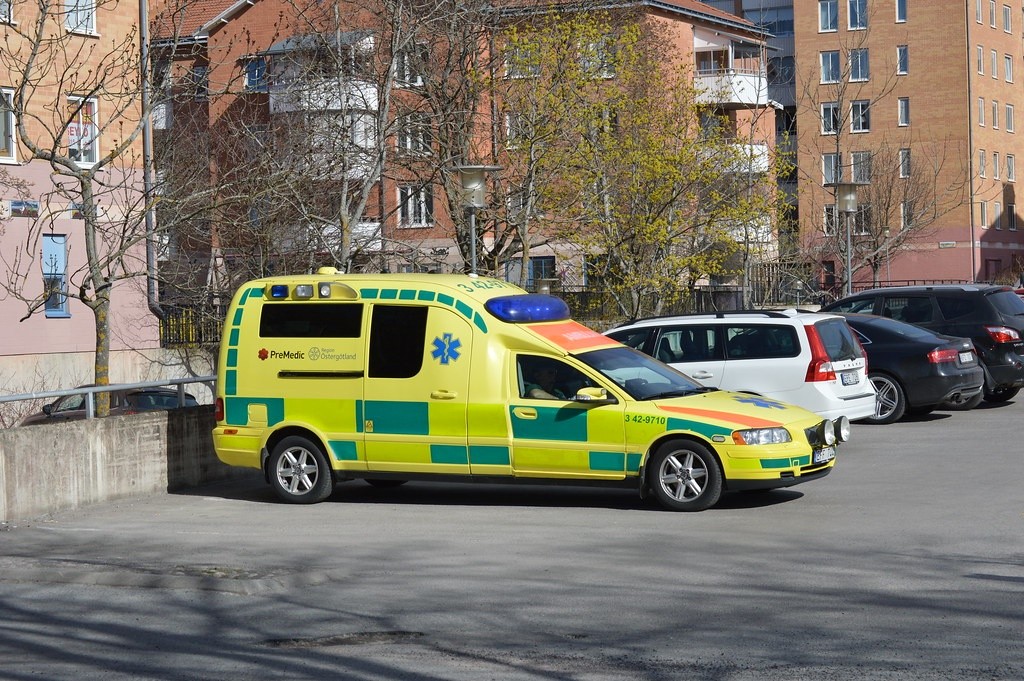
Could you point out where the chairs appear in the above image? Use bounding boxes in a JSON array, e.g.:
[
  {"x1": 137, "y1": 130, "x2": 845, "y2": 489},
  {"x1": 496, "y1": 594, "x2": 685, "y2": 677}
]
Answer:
[
  {"x1": 884, "y1": 307, "x2": 892, "y2": 317},
  {"x1": 658, "y1": 330, "x2": 698, "y2": 363},
  {"x1": 899, "y1": 305, "x2": 913, "y2": 323}
]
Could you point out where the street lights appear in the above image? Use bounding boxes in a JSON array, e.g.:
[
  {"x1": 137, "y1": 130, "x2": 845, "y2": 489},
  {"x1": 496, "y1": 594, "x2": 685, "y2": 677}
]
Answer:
[
  {"x1": 878, "y1": 226, "x2": 894, "y2": 286},
  {"x1": 443, "y1": 165, "x2": 504, "y2": 275},
  {"x1": 792, "y1": 280, "x2": 805, "y2": 308},
  {"x1": 822, "y1": 181, "x2": 873, "y2": 297}
]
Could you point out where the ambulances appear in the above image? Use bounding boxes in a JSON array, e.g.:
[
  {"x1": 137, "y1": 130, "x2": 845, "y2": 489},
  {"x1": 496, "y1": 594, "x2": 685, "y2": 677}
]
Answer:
[{"x1": 208, "y1": 266, "x2": 853, "y2": 515}]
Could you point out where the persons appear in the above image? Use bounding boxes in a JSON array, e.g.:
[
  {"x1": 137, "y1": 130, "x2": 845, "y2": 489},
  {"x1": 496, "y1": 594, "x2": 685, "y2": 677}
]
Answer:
[{"x1": 526, "y1": 365, "x2": 566, "y2": 400}]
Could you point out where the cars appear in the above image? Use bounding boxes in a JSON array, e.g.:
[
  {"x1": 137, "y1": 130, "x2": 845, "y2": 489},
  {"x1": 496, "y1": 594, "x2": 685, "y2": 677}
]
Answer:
[
  {"x1": 826, "y1": 312, "x2": 984, "y2": 424},
  {"x1": 812, "y1": 284, "x2": 1024, "y2": 411},
  {"x1": 599, "y1": 306, "x2": 877, "y2": 422}
]
